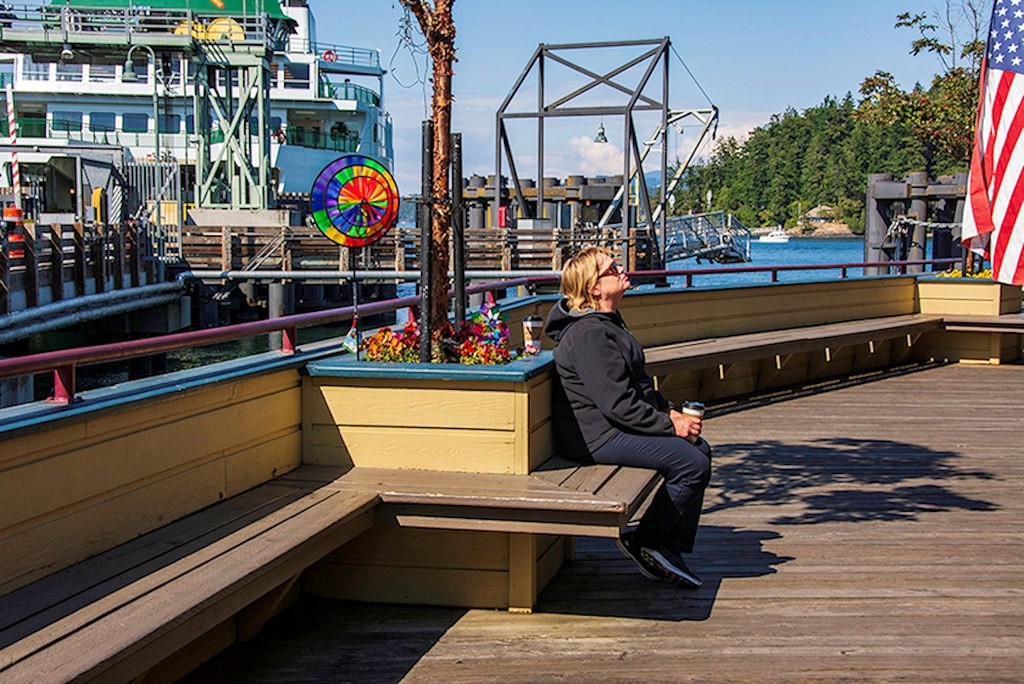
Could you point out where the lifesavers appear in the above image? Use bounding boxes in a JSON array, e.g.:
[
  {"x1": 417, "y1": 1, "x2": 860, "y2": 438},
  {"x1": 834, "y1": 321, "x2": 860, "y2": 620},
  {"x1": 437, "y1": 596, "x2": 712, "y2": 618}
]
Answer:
[{"x1": 324, "y1": 51, "x2": 334, "y2": 61}]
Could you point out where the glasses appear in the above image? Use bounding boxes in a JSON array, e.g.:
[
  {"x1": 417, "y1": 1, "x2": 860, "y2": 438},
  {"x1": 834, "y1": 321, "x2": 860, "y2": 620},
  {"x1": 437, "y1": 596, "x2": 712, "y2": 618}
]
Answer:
[{"x1": 599, "y1": 260, "x2": 619, "y2": 278}]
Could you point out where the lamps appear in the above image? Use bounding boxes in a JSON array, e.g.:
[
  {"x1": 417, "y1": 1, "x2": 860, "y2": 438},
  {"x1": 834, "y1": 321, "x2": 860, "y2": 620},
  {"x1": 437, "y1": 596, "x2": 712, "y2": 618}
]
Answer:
[
  {"x1": 60, "y1": 44, "x2": 75, "y2": 61},
  {"x1": 594, "y1": 123, "x2": 610, "y2": 144}
]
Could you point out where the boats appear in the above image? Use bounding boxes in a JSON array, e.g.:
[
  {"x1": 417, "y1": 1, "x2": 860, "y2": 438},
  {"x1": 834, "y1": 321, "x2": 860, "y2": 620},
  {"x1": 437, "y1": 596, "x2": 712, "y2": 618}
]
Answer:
[
  {"x1": 1, "y1": 1, "x2": 395, "y2": 320},
  {"x1": 758, "y1": 224, "x2": 794, "y2": 243}
]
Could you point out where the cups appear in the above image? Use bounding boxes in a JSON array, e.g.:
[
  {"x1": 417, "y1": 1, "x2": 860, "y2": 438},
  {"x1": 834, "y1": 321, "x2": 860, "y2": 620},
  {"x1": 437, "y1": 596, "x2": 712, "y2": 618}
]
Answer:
[
  {"x1": 522, "y1": 315, "x2": 544, "y2": 355},
  {"x1": 681, "y1": 401, "x2": 705, "y2": 442}
]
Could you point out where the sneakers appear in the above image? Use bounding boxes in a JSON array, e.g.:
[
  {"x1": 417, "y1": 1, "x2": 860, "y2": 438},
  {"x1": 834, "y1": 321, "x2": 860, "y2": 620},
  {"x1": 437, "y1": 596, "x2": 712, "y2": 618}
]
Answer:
[
  {"x1": 640, "y1": 544, "x2": 704, "y2": 589},
  {"x1": 616, "y1": 530, "x2": 665, "y2": 580}
]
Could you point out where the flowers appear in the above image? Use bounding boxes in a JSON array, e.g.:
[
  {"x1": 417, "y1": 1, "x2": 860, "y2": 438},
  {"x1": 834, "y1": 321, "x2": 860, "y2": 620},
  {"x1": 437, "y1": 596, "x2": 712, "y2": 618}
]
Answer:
[
  {"x1": 361, "y1": 302, "x2": 512, "y2": 365},
  {"x1": 934, "y1": 267, "x2": 994, "y2": 280}
]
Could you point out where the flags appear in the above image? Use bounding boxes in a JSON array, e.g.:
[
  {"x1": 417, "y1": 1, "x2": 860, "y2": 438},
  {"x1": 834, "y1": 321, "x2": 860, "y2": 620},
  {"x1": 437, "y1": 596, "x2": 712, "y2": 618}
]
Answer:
[{"x1": 961, "y1": 0, "x2": 1024, "y2": 285}]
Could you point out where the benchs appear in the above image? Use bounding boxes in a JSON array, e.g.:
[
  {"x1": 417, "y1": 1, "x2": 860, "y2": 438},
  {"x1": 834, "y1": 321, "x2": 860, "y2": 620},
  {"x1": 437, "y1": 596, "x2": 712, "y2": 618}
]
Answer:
[
  {"x1": 642, "y1": 311, "x2": 1024, "y2": 416},
  {"x1": 0, "y1": 456, "x2": 667, "y2": 684}
]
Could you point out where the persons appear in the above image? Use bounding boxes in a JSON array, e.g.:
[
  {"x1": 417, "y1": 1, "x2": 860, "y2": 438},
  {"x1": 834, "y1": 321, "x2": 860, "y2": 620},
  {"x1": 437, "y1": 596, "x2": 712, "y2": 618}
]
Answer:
[{"x1": 544, "y1": 247, "x2": 712, "y2": 589}]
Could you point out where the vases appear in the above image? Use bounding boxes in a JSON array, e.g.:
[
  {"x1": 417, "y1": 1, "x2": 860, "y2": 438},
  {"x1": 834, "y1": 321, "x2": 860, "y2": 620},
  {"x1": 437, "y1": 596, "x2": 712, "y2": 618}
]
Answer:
[
  {"x1": 916, "y1": 275, "x2": 1023, "y2": 366},
  {"x1": 294, "y1": 349, "x2": 557, "y2": 616}
]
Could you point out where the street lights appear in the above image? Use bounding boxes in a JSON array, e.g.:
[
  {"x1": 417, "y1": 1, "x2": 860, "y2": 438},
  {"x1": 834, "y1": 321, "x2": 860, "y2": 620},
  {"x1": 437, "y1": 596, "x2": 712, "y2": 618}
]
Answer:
[{"x1": 121, "y1": 44, "x2": 163, "y2": 257}]
